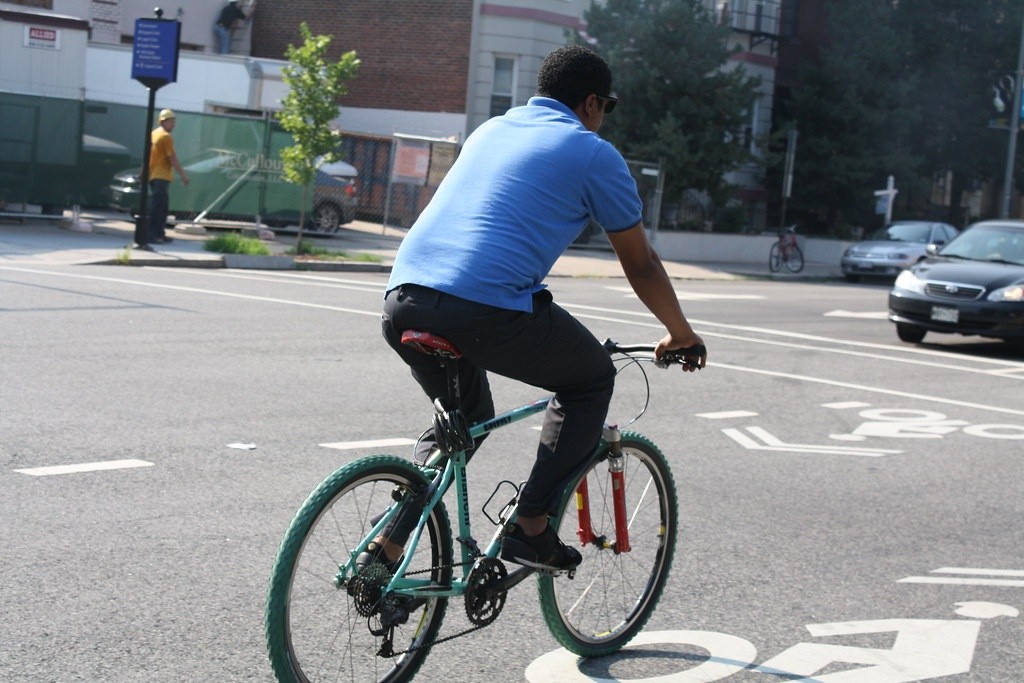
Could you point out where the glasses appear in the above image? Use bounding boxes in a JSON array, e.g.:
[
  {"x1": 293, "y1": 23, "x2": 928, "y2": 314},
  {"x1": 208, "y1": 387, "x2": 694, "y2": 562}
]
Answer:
[{"x1": 590, "y1": 94, "x2": 618, "y2": 113}]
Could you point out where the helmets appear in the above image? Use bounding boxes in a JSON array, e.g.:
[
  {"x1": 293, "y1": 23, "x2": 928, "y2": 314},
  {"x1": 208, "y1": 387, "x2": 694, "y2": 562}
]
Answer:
[{"x1": 158, "y1": 109, "x2": 175, "y2": 125}]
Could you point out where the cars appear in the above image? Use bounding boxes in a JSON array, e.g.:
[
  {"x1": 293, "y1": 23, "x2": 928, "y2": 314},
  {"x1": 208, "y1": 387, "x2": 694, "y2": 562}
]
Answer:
[
  {"x1": 888, "y1": 219, "x2": 1023, "y2": 360},
  {"x1": 840, "y1": 221, "x2": 962, "y2": 285},
  {"x1": 109, "y1": 145, "x2": 357, "y2": 238}
]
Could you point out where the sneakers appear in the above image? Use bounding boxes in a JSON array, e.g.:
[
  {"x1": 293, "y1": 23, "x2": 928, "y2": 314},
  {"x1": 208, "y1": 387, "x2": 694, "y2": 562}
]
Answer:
[
  {"x1": 356, "y1": 542, "x2": 404, "y2": 575},
  {"x1": 500, "y1": 520, "x2": 582, "y2": 571}
]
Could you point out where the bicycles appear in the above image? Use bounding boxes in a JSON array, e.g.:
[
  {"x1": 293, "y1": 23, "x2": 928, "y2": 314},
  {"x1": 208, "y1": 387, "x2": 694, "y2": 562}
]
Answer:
[
  {"x1": 263, "y1": 329, "x2": 706, "y2": 683},
  {"x1": 769, "y1": 224, "x2": 805, "y2": 273}
]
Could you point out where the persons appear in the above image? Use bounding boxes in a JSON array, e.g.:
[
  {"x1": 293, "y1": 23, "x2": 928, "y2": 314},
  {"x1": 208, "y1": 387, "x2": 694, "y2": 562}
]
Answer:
[
  {"x1": 135, "y1": 107, "x2": 189, "y2": 244},
  {"x1": 214, "y1": 1, "x2": 256, "y2": 56},
  {"x1": 352, "y1": 44, "x2": 706, "y2": 591}
]
